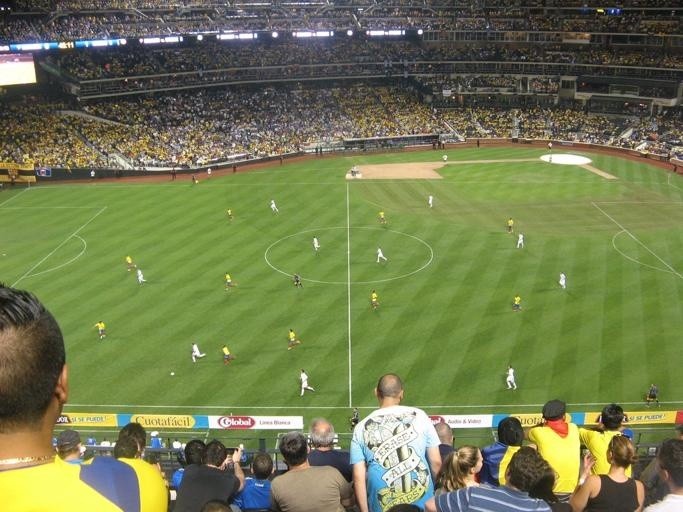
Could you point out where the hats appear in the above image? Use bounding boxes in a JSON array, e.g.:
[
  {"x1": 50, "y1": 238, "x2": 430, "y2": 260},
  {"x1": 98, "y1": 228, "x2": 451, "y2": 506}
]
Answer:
[
  {"x1": 56, "y1": 430, "x2": 80, "y2": 450},
  {"x1": 542, "y1": 400, "x2": 566, "y2": 420}
]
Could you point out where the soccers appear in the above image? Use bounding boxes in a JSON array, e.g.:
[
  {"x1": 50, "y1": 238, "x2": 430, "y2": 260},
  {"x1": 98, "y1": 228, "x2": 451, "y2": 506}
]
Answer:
[{"x1": 170, "y1": 371, "x2": 175, "y2": 376}]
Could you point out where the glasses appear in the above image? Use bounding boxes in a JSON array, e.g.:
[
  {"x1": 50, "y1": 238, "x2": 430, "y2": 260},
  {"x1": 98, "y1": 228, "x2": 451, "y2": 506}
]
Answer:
[{"x1": 611, "y1": 434, "x2": 630, "y2": 448}]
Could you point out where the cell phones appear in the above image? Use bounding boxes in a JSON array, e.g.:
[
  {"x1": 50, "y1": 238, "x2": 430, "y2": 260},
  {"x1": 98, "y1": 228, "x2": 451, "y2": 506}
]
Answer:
[{"x1": 225, "y1": 447, "x2": 239, "y2": 455}]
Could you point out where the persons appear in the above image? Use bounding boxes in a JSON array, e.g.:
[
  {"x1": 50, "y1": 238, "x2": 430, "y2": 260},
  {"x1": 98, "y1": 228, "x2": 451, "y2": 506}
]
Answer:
[
  {"x1": 125, "y1": 254, "x2": 135, "y2": 269},
  {"x1": 55, "y1": 408, "x2": 422, "y2": 511},
  {"x1": 504, "y1": 364, "x2": 517, "y2": 389},
  {"x1": 512, "y1": 294, "x2": 522, "y2": 311},
  {"x1": 221, "y1": 343, "x2": 238, "y2": 366},
  {"x1": 645, "y1": 383, "x2": 660, "y2": 408},
  {"x1": 287, "y1": 328, "x2": 301, "y2": 352},
  {"x1": 292, "y1": 273, "x2": 304, "y2": 290},
  {"x1": 427, "y1": 194, "x2": 433, "y2": 208},
  {"x1": 558, "y1": 271, "x2": 566, "y2": 290},
  {"x1": 225, "y1": 207, "x2": 233, "y2": 219},
  {"x1": 349, "y1": 374, "x2": 442, "y2": 512},
  {"x1": 0, "y1": 280, "x2": 168, "y2": 512},
  {"x1": 93, "y1": 321, "x2": 108, "y2": 339},
  {"x1": 507, "y1": 217, "x2": 513, "y2": 232},
  {"x1": 516, "y1": 233, "x2": 524, "y2": 249},
  {"x1": 1, "y1": 1, "x2": 682, "y2": 184},
  {"x1": 134, "y1": 267, "x2": 146, "y2": 286},
  {"x1": 375, "y1": 247, "x2": 388, "y2": 264},
  {"x1": 377, "y1": 209, "x2": 387, "y2": 226},
  {"x1": 221, "y1": 271, "x2": 238, "y2": 293},
  {"x1": 310, "y1": 235, "x2": 322, "y2": 252},
  {"x1": 425, "y1": 400, "x2": 682, "y2": 511},
  {"x1": 268, "y1": 198, "x2": 280, "y2": 216},
  {"x1": 298, "y1": 370, "x2": 315, "y2": 398},
  {"x1": 190, "y1": 343, "x2": 206, "y2": 365},
  {"x1": 370, "y1": 290, "x2": 378, "y2": 310}
]
